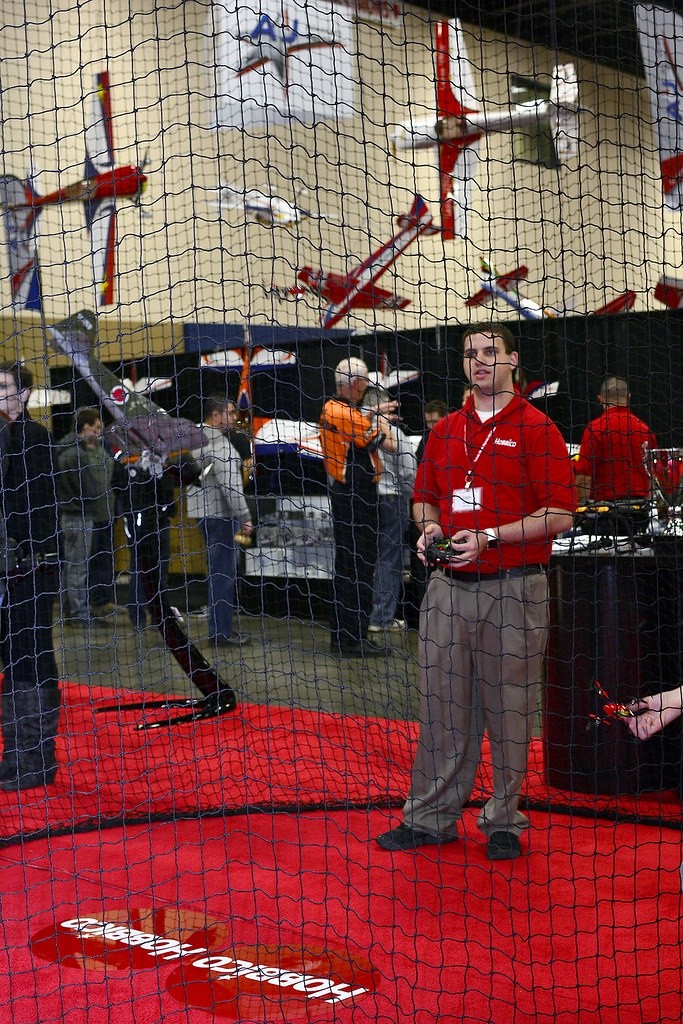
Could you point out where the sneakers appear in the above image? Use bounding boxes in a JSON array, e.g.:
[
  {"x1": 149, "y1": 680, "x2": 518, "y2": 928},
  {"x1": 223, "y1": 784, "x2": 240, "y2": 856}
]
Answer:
[
  {"x1": 377, "y1": 822, "x2": 458, "y2": 849},
  {"x1": 488, "y1": 831, "x2": 520, "y2": 859}
]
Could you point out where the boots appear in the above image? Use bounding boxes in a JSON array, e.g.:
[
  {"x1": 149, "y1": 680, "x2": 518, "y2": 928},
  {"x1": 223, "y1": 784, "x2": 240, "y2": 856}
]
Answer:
[{"x1": 0, "y1": 678, "x2": 61, "y2": 791}]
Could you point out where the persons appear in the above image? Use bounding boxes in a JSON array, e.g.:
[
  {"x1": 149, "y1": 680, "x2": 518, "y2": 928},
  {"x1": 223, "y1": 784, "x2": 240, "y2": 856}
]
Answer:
[
  {"x1": 461, "y1": 384, "x2": 472, "y2": 407},
  {"x1": 409, "y1": 400, "x2": 448, "y2": 631},
  {"x1": 623, "y1": 686, "x2": 683, "y2": 740},
  {"x1": 185, "y1": 397, "x2": 253, "y2": 646},
  {"x1": 577, "y1": 376, "x2": 658, "y2": 537},
  {"x1": 113, "y1": 452, "x2": 200, "y2": 630},
  {"x1": 0, "y1": 360, "x2": 60, "y2": 788},
  {"x1": 322, "y1": 357, "x2": 404, "y2": 660},
  {"x1": 375, "y1": 322, "x2": 576, "y2": 858},
  {"x1": 58, "y1": 407, "x2": 129, "y2": 628},
  {"x1": 362, "y1": 390, "x2": 420, "y2": 632}
]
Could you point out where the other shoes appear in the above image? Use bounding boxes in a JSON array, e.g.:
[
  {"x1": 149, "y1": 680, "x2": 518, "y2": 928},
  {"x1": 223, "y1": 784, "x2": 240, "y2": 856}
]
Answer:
[
  {"x1": 341, "y1": 646, "x2": 391, "y2": 657},
  {"x1": 368, "y1": 619, "x2": 405, "y2": 631},
  {"x1": 90, "y1": 601, "x2": 127, "y2": 617},
  {"x1": 331, "y1": 640, "x2": 376, "y2": 653},
  {"x1": 208, "y1": 633, "x2": 253, "y2": 646}
]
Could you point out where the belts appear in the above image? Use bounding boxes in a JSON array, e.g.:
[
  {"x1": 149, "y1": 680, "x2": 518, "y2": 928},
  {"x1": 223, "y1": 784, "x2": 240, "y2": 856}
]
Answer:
[{"x1": 436, "y1": 564, "x2": 544, "y2": 582}]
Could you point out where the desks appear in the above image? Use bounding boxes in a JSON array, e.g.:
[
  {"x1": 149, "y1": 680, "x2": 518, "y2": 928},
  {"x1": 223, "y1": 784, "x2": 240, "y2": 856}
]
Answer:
[
  {"x1": 241, "y1": 454, "x2": 427, "y2": 630},
  {"x1": 543, "y1": 536, "x2": 682, "y2": 795}
]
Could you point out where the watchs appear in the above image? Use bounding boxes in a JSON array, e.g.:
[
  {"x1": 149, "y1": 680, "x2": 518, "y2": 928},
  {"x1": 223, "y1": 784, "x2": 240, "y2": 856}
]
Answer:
[{"x1": 485, "y1": 529, "x2": 498, "y2": 550}]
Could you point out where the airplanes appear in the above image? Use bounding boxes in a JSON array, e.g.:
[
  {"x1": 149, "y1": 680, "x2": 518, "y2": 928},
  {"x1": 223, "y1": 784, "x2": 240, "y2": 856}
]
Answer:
[
  {"x1": 231, "y1": 7, "x2": 349, "y2": 99},
  {"x1": 11, "y1": 70, "x2": 149, "y2": 308},
  {"x1": 462, "y1": 255, "x2": 639, "y2": 323},
  {"x1": 191, "y1": 323, "x2": 585, "y2": 497},
  {"x1": 587, "y1": 677, "x2": 648, "y2": 729},
  {"x1": 391, "y1": 17, "x2": 582, "y2": 241},
  {"x1": 288, "y1": 193, "x2": 444, "y2": 330},
  {"x1": 44, "y1": 309, "x2": 209, "y2": 493},
  {"x1": 209, "y1": 180, "x2": 340, "y2": 231}
]
